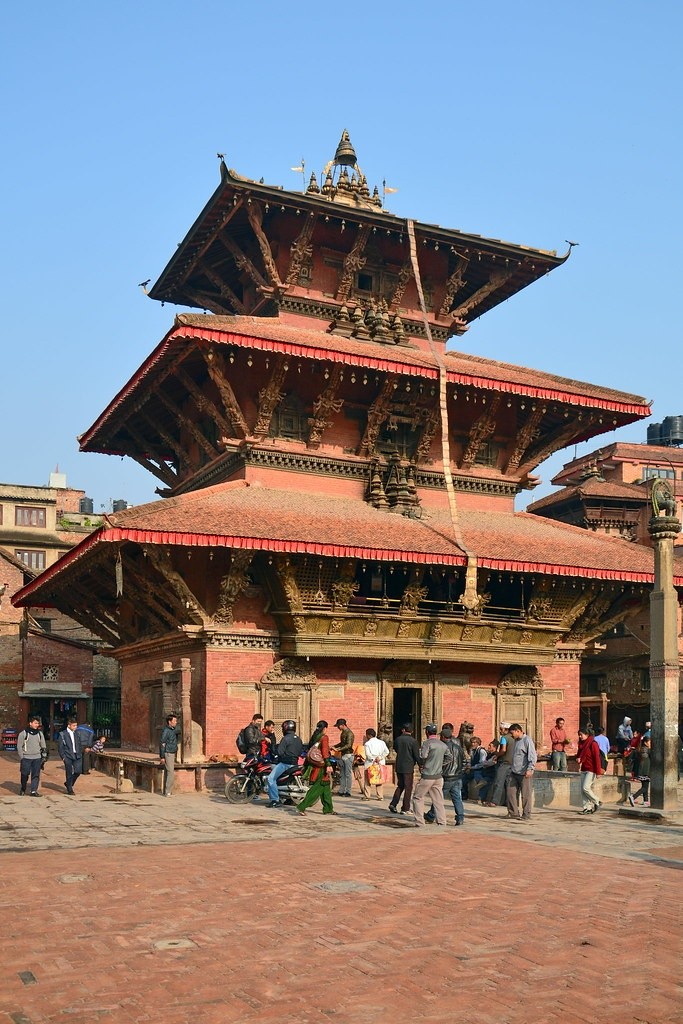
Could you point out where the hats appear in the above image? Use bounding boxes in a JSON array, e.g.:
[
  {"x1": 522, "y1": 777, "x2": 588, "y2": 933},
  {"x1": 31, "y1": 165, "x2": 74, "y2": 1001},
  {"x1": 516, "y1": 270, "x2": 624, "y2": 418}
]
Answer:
[
  {"x1": 423, "y1": 725, "x2": 437, "y2": 734},
  {"x1": 500, "y1": 722, "x2": 512, "y2": 729},
  {"x1": 439, "y1": 729, "x2": 452, "y2": 738},
  {"x1": 334, "y1": 719, "x2": 346, "y2": 727}
]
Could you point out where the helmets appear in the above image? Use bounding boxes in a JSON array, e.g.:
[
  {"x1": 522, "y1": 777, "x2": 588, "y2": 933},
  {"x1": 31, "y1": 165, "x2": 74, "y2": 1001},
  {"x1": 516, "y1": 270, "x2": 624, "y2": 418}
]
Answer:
[{"x1": 281, "y1": 720, "x2": 297, "y2": 736}]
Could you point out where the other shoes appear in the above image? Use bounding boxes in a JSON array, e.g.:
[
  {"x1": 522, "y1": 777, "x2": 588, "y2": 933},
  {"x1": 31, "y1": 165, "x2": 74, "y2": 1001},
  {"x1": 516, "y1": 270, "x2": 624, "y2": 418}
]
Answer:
[
  {"x1": 629, "y1": 796, "x2": 635, "y2": 807},
  {"x1": 578, "y1": 809, "x2": 592, "y2": 815},
  {"x1": 336, "y1": 792, "x2": 344, "y2": 796},
  {"x1": 67, "y1": 788, "x2": 76, "y2": 795},
  {"x1": 325, "y1": 811, "x2": 338, "y2": 815},
  {"x1": 389, "y1": 805, "x2": 397, "y2": 813},
  {"x1": 295, "y1": 807, "x2": 307, "y2": 816},
  {"x1": 502, "y1": 814, "x2": 520, "y2": 819},
  {"x1": 361, "y1": 796, "x2": 370, "y2": 801},
  {"x1": 20, "y1": 789, "x2": 25, "y2": 796},
  {"x1": 518, "y1": 816, "x2": 530, "y2": 821},
  {"x1": 64, "y1": 782, "x2": 68, "y2": 787},
  {"x1": 378, "y1": 797, "x2": 383, "y2": 801},
  {"x1": 253, "y1": 795, "x2": 262, "y2": 801},
  {"x1": 593, "y1": 801, "x2": 603, "y2": 814},
  {"x1": 84, "y1": 772, "x2": 90, "y2": 775},
  {"x1": 30, "y1": 792, "x2": 43, "y2": 797},
  {"x1": 462, "y1": 797, "x2": 468, "y2": 800},
  {"x1": 642, "y1": 801, "x2": 649, "y2": 806},
  {"x1": 424, "y1": 812, "x2": 434, "y2": 823},
  {"x1": 400, "y1": 809, "x2": 414, "y2": 815},
  {"x1": 345, "y1": 793, "x2": 351, "y2": 797},
  {"x1": 163, "y1": 793, "x2": 176, "y2": 797},
  {"x1": 266, "y1": 800, "x2": 283, "y2": 808},
  {"x1": 473, "y1": 780, "x2": 487, "y2": 790},
  {"x1": 473, "y1": 800, "x2": 486, "y2": 804},
  {"x1": 455, "y1": 821, "x2": 463, "y2": 826},
  {"x1": 284, "y1": 799, "x2": 293, "y2": 805},
  {"x1": 484, "y1": 802, "x2": 496, "y2": 807}
]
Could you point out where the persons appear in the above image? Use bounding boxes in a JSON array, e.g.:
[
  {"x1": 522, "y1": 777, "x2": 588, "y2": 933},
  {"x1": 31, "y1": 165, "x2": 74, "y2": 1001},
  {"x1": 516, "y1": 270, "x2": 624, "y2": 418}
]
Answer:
[
  {"x1": 629, "y1": 736, "x2": 651, "y2": 807},
  {"x1": 616, "y1": 716, "x2": 652, "y2": 758},
  {"x1": 90, "y1": 735, "x2": 106, "y2": 771},
  {"x1": 550, "y1": 718, "x2": 570, "y2": 772},
  {"x1": 485, "y1": 723, "x2": 538, "y2": 821},
  {"x1": 58, "y1": 717, "x2": 83, "y2": 795},
  {"x1": 260, "y1": 720, "x2": 275, "y2": 756},
  {"x1": 17, "y1": 716, "x2": 47, "y2": 797},
  {"x1": 678, "y1": 731, "x2": 683, "y2": 780},
  {"x1": 412, "y1": 723, "x2": 465, "y2": 826},
  {"x1": 244, "y1": 714, "x2": 271, "y2": 801},
  {"x1": 159, "y1": 714, "x2": 178, "y2": 798},
  {"x1": 361, "y1": 728, "x2": 390, "y2": 801},
  {"x1": 594, "y1": 726, "x2": 610, "y2": 776},
  {"x1": 74, "y1": 720, "x2": 94, "y2": 775},
  {"x1": 329, "y1": 719, "x2": 354, "y2": 797},
  {"x1": 461, "y1": 737, "x2": 500, "y2": 805},
  {"x1": 389, "y1": 723, "x2": 424, "y2": 815},
  {"x1": 265, "y1": 720, "x2": 303, "y2": 808},
  {"x1": 295, "y1": 720, "x2": 338, "y2": 817},
  {"x1": 574, "y1": 728, "x2": 605, "y2": 815}
]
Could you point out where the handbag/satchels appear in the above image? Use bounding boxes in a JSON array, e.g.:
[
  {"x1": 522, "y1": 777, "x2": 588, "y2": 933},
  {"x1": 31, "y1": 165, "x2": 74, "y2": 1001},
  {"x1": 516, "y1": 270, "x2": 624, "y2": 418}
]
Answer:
[
  {"x1": 306, "y1": 741, "x2": 325, "y2": 767},
  {"x1": 367, "y1": 761, "x2": 388, "y2": 785}
]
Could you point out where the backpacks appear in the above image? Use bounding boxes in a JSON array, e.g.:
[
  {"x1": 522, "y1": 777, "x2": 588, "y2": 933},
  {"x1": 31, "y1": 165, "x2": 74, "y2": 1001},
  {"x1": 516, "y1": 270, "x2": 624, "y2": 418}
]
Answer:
[
  {"x1": 590, "y1": 740, "x2": 608, "y2": 772},
  {"x1": 236, "y1": 726, "x2": 256, "y2": 754}
]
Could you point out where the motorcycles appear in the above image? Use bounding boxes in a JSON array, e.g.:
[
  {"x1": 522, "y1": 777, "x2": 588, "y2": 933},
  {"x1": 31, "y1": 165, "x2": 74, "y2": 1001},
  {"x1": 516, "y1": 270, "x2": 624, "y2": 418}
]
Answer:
[
  {"x1": 224, "y1": 741, "x2": 321, "y2": 807},
  {"x1": 328, "y1": 755, "x2": 367, "y2": 794}
]
